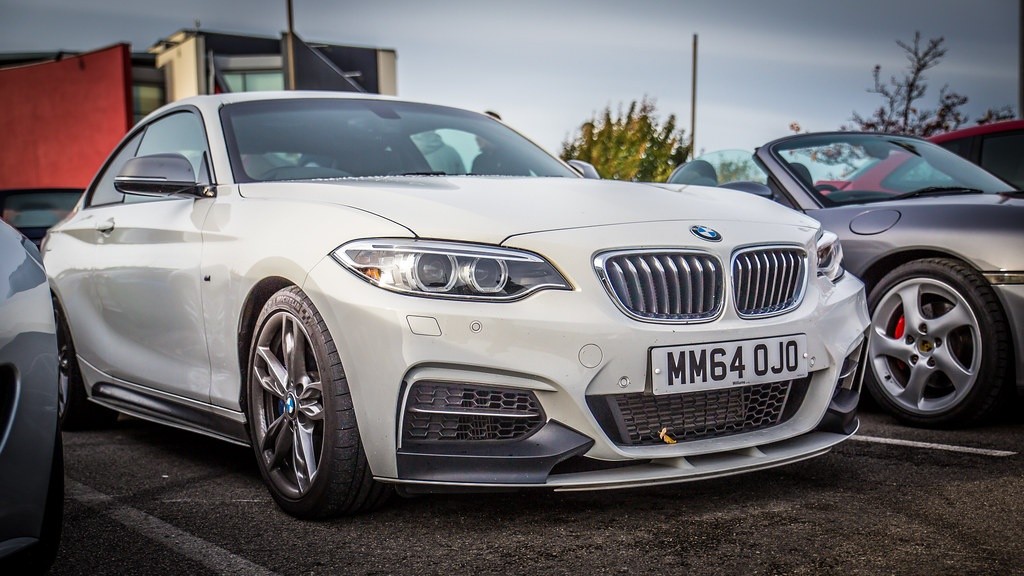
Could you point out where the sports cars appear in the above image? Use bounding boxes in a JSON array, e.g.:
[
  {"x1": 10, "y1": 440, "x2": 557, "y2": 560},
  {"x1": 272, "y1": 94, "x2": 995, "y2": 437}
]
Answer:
[{"x1": 665, "y1": 129, "x2": 1024, "y2": 428}]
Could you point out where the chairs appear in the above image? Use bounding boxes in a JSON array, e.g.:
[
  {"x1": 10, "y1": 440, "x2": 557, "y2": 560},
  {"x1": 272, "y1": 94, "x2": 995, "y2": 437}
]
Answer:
[{"x1": 767, "y1": 162, "x2": 814, "y2": 210}]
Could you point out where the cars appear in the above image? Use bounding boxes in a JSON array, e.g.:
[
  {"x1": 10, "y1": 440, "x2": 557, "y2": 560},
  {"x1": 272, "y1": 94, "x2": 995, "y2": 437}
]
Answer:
[
  {"x1": 31, "y1": 89, "x2": 874, "y2": 521},
  {"x1": 811, "y1": 119, "x2": 1024, "y2": 200},
  {"x1": 0, "y1": 218, "x2": 73, "y2": 576}
]
